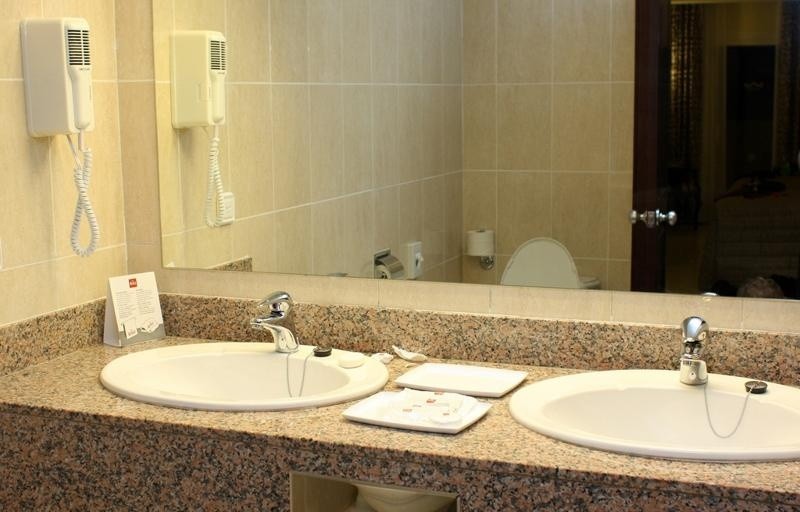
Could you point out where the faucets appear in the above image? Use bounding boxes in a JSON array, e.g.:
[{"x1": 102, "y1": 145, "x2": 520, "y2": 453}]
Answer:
[
  {"x1": 250, "y1": 290, "x2": 298, "y2": 352},
  {"x1": 679, "y1": 315, "x2": 708, "y2": 385}
]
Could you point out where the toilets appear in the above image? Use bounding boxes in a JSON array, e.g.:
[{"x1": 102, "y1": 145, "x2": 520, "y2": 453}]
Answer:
[{"x1": 498, "y1": 237, "x2": 601, "y2": 287}]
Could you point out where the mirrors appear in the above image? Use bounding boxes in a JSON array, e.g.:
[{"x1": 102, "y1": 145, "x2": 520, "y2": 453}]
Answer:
[{"x1": 153, "y1": 1, "x2": 799, "y2": 299}]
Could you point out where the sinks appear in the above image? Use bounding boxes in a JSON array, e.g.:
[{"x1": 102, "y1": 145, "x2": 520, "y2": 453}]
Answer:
[
  {"x1": 101, "y1": 339, "x2": 388, "y2": 412},
  {"x1": 510, "y1": 367, "x2": 796, "y2": 463}
]
